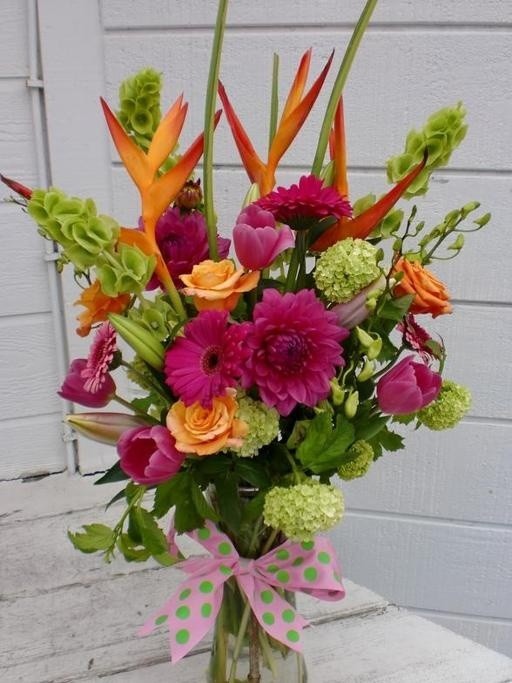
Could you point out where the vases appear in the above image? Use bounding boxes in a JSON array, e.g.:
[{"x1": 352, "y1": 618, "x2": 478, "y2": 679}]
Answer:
[
  {"x1": 3, "y1": 3, "x2": 492, "y2": 682},
  {"x1": 200, "y1": 485, "x2": 305, "y2": 680}
]
